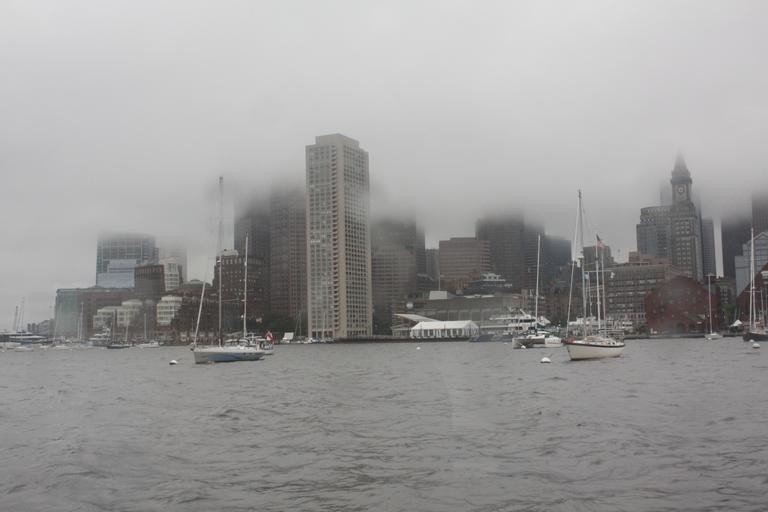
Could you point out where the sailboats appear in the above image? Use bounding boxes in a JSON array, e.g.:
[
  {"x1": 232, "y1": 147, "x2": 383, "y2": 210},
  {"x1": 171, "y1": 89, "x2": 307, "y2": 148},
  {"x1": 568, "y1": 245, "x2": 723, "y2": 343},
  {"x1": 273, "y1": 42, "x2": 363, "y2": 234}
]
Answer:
[
  {"x1": 512, "y1": 234, "x2": 624, "y2": 350},
  {"x1": 705, "y1": 275, "x2": 721, "y2": 340},
  {"x1": 560, "y1": 190, "x2": 627, "y2": 361},
  {"x1": 727, "y1": 228, "x2": 767, "y2": 349},
  {"x1": 0, "y1": 306, "x2": 160, "y2": 353},
  {"x1": 189, "y1": 231, "x2": 266, "y2": 365}
]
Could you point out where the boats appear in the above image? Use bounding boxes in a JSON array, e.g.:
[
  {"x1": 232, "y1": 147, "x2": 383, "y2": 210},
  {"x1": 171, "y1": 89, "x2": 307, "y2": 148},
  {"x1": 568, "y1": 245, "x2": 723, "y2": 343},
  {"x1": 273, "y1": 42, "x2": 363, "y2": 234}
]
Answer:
[
  {"x1": 224, "y1": 332, "x2": 275, "y2": 355},
  {"x1": 279, "y1": 335, "x2": 334, "y2": 345}
]
